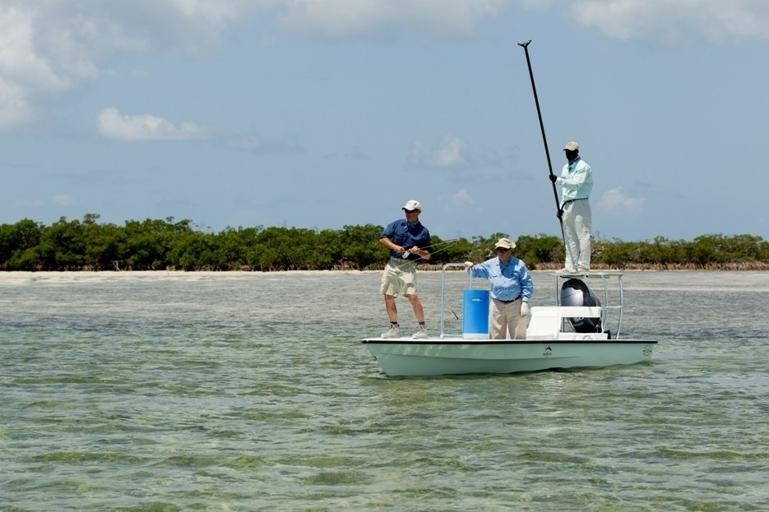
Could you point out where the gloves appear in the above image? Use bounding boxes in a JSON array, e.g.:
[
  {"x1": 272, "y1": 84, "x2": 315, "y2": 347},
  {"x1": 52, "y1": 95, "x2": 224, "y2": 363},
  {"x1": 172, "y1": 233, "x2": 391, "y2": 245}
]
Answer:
[{"x1": 520, "y1": 302, "x2": 530, "y2": 318}]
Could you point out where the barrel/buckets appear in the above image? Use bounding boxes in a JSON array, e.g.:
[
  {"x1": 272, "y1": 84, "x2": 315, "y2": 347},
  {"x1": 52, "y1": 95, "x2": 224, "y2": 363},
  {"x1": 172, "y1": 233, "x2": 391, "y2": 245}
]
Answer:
[{"x1": 461, "y1": 288, "x2": 490, "y2": 340}]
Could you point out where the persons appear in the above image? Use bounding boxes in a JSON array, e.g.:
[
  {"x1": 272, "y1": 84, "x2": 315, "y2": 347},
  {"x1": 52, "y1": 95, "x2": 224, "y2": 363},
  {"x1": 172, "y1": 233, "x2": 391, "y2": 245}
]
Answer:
[
  {"x1": 549, "y1": 141, "x2": 593, "y2": 273},
  {"x1": 465, "y1": 238, "x2": 533, "y2": 339},
  {"x1": 379, "y1": 200, "x2": 433, "y2": 338}
]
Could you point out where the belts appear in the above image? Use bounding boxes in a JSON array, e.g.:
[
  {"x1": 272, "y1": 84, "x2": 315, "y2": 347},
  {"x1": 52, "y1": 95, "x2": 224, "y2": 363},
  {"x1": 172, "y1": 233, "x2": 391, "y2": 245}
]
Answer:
[{"x1": 561, "y1": 198, "x2": 588, "y2": 209}]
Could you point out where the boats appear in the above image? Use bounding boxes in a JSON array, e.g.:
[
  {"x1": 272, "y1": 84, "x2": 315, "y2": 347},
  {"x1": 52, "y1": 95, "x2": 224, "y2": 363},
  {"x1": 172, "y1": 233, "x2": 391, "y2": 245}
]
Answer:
[{"x1": 360, "y1": 271, "x2": 658, "y2": 376}]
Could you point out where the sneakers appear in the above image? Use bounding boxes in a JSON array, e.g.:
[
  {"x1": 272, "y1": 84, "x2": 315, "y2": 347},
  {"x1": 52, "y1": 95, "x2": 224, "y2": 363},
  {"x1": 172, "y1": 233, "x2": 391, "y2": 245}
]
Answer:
[
  {"x1": 381, "y1": 327, "x2": 401, "y2": 339},
  {"x1": 412, "y1": 329, "x2": 429, "y2": 339}
]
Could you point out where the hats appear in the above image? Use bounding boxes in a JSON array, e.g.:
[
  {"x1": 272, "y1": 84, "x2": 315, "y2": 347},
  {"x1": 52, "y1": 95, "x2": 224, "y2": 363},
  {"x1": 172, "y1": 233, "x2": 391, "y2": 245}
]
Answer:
[
  {"x1": 401, "y1": 199, "x2": 423, "y2": 213},
  {"x1": 494, "y1": 238, "x2": 517, "y2": 251},
  {"x1": 562, "y1": 141, "x2": 580, "y2": 153}
]
